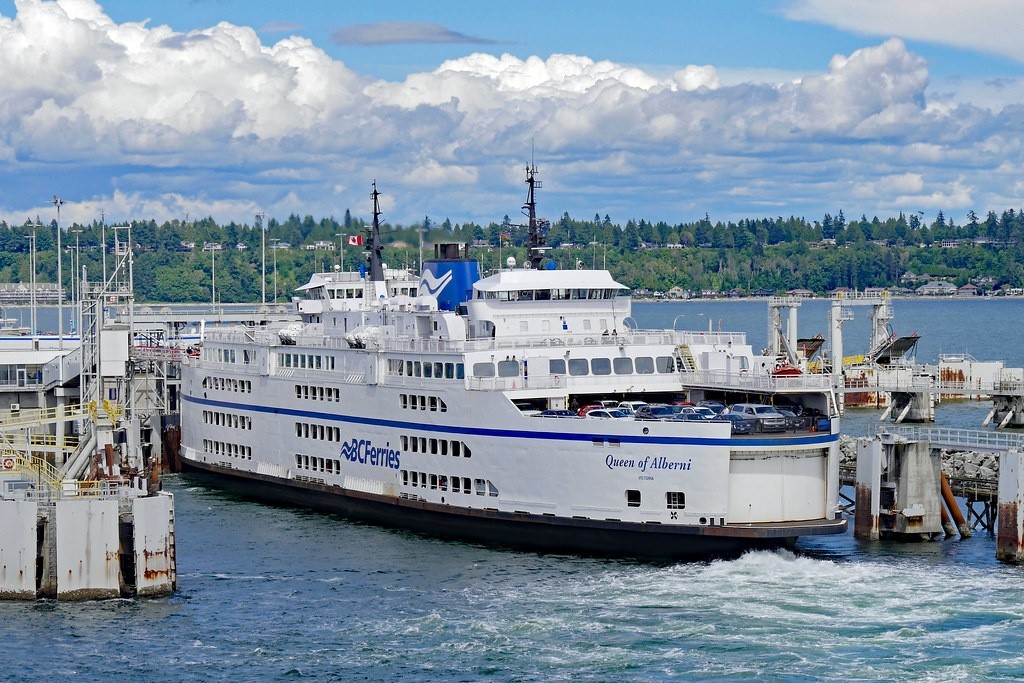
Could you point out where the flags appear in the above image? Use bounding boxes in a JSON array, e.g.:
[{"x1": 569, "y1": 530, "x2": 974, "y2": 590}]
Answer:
[{"x1": 346, "y1": 235, "x2": 363, "y2": 246}]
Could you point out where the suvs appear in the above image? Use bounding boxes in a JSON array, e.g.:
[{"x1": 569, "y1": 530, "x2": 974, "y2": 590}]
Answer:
[{"x1": 730, "y1": 403, "x2": 784, "y2": 432}]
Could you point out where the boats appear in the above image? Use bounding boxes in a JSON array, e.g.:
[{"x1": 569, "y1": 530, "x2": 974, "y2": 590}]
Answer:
[
  {"x1": 174, "y1": 136, "x2": 851, "y2": 550},
  {"x1": 0, "y1": 305, "x2": 200, "y2": 349}
]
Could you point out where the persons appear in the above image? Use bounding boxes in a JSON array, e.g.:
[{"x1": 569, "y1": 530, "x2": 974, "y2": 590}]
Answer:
[
  {"x1": 186, "y1": 346, "x2": 200, "y2": 354},
  {"x1": 601, "y1": 329, "x2": 617, "y2": 344},
  {"x1": 571, "y1": 398, "x2": 578, "y2": 413},
  {"x1": 439, "y1": 336, "x2": 444, "y2": 350},
  {"x1": 789, "y1": 405, "x2": 803, "y2": 417}
]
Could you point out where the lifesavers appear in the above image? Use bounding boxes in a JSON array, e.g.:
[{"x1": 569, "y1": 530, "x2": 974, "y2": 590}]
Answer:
[{"x1": 4, "y1": 458, "x2": 14, "y2": 468}]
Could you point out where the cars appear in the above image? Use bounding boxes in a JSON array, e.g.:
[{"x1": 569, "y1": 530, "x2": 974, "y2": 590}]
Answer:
[{"x1": 512, "y1": 400, "x2": 818, "y2": 432}]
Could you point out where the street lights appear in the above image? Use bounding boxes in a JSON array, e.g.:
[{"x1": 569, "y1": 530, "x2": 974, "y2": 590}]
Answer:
[
  {"x1": 73, "y1": 229, "x2": 84, "y2": 335},
  {"x1": 206, "y1": 242, "x2": 218, "y2": 309},
  {"x1": 43, "y1": 199, "x2": 73, "y2": 350},
  {"x1": 99, "y1": 210, "x2": 110, "y2": 314},
  {"x1": 25, "y1": 235, "x2": 37, "y2": 336},
  {"x1": 335, "y1": 234, "x2": 347, "y2": 273},
  {"x1": 269, "y1": 238, "x2": 281, "y2": 304},
  {"x1": 27, "y1": 222, "x2": 43, "y2": 336},
  {"x1": 65, "y1": 245, "x2": 75, "y2": 334},
  {"x1": 257, "y1": 212, "x2": 271, "y2": 303}
]
